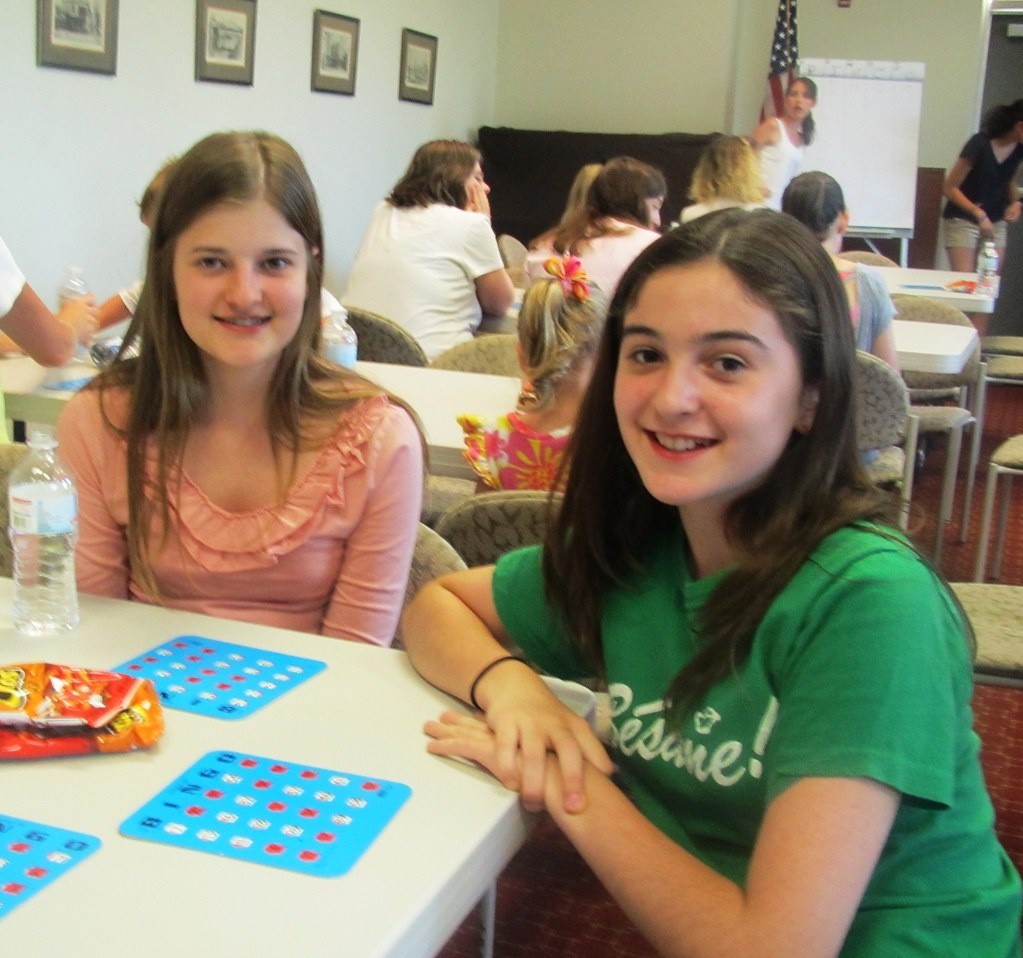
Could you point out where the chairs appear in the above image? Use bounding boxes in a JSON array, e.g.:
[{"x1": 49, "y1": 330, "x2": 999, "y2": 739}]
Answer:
[{"x1": 0, "y1": 251, "x2": 1023, "y2": 958}]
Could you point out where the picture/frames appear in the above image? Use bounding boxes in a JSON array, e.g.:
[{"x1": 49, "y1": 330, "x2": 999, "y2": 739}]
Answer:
[
  {"x1": 195, "y1": 0, "x2": 258, "y2": 86},
  {"x1": 312, "y1": 9, "x2": 360, "y2": 95},
  {"x1": 400, "y1": 26, "x2": 438, "y2": 106},
  {"x1": 37, "y1": 0, "x2": 119, "y2": 76}
]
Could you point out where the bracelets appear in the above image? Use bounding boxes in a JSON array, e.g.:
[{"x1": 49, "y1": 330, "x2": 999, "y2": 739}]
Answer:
[{"x1": 468, "y1": 656, "x2": 527, "y2": 714}]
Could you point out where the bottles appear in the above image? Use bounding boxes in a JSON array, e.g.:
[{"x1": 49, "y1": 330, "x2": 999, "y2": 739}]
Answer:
[
  {"x1": 6, "y1": 431, "x2": 80, "y2": 635},
  {"x1": 322, "y1": 309, "x2": 358, "y2": 370},
  {"x1": 976, "y1": 241, "x2": 999, "y2": 296},
  {"x1": 58, "y1": 265, "x2": 90, "y2": 363}
]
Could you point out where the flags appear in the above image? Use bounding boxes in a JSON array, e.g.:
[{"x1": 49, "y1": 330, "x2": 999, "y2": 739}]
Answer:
[{"x1": 759, "y1": 0, "x2": 800, "y2": 124}]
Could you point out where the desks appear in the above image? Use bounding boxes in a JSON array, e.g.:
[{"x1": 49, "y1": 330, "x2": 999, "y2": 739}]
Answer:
[
  {"x1": 0, "y1": 335, "x2": 526, "y2": 483},
  {"x1": 508, "y1": 281, "x2": 979, "y2": 375},
  {"x1": 1, "y1": 575, "x2": 596, "y2": 958},
  {"x1": 861, "y1": 262, "x2": 1002, "y2": 313}
]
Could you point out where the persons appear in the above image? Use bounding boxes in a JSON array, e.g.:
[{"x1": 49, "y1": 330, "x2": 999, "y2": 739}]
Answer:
[
  {"x1": 782, "y1": 171, "x2": 898, "y2": 467},
  {"x1": 93, "y1": 160, "x2": 358, "y2": 368},
  {"x1": 341, "y1": 139, "x2": 515, "y2": 366},
  {"x1": 943, "y1": 97, "x2": 1023, "y2": 337},
  {"x1": 0, "y1": 235, "x2": 99, "y2": 369},
  {"x1": 457, "y1": 257, "x2": 612, "y2": 493},
  {"x1": 681, "y1": 134, "x2": 769, "y2": 224},
  {"x1": 52, "y1": 130, "x2": 431, "y2": 652},
  {"x1": 525, "y1": 154, "x2": 668, "y2": 304},
  {"x1": 399, "y1": 207, "x2": 1023, "y2": 958},
  {"x1": 752, "y1": 76, "x2": 818, "y2": 215}
]
